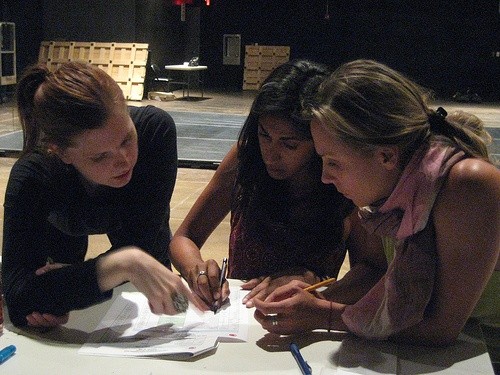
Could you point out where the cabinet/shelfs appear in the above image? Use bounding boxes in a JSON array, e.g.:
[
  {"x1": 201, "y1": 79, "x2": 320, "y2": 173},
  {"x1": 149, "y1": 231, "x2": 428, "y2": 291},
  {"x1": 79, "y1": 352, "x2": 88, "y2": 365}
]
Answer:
[{"x1": 0, "y1": 22, "x2": 16, "y2": 85}]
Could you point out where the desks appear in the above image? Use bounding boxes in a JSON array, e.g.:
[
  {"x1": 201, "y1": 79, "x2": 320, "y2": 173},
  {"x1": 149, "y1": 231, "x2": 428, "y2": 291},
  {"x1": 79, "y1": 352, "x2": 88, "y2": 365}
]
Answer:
[
  {"x1": 165, "y1": 64, "x2": 208, "y2": 100},
  {"x1": 0, "y1": 277, "x2": 496, "y2": 375}
]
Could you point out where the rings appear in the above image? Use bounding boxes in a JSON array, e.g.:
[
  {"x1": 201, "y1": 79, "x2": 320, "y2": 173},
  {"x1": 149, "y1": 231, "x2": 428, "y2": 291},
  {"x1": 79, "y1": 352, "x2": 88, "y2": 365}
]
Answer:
[
  {"x1": 172, "y1": 292, "x2": 189, "y2": 312},
  {"x1": 271, "y1": 315, "x2": 279, "y2": 327},
  {"x1": 196, "y1": 270, "x2": 208, "y2": 281}
]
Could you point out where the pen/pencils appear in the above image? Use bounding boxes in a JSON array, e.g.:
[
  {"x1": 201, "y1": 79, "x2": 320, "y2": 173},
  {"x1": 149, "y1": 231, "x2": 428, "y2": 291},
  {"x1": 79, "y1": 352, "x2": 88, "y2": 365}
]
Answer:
[
  {"x1": 304, "y1": 278, "x2": 335, "y2": 293},
  {"x1": 214, "y1": 257, "x2": 228, "y2": 314},
  {"x1": 289, "y1": 341, "x2": 312, "y2": 375},
  {"x1": 0, "y1": 345, "x2": 16, "y2": 364}
]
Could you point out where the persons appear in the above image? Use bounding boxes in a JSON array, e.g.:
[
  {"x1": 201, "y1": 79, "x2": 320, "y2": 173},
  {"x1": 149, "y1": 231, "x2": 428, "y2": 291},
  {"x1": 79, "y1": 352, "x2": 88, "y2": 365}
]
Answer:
[
  {"x1": 167, "y1": 60, "x2": 388, "y2": 312},
  {"x1": 254, "y1": 59, "x2": 500, "y2": 375},
  {"x1": 0, "y1": 59, "x2": 206, "y2": 331}
]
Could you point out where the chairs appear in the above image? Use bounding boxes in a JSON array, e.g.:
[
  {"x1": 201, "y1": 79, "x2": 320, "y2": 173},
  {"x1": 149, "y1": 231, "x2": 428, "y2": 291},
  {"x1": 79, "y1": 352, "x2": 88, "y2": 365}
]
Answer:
[{"x1": 151, "y1": 64, "x2": 173, "y2": 92}]
